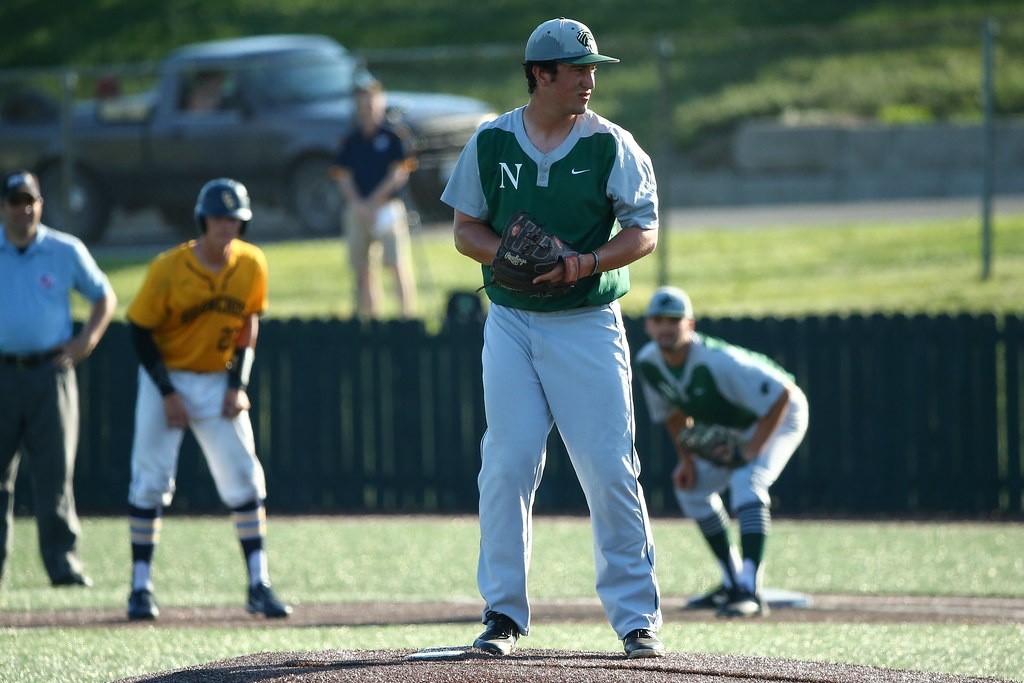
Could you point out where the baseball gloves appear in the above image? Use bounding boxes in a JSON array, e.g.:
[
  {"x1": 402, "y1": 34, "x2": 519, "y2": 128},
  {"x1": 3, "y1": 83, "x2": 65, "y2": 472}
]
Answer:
[
  {"x1": 475, "y1": 209, "x2": 581, "y2": 297},
  {"x1": 676, "y1": 423, "x2": 748, "y2": 469}
]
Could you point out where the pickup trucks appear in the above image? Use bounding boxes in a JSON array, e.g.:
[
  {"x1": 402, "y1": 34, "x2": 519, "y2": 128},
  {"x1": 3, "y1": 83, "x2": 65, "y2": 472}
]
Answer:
[{"x1": 0, "y1": 33, "x2": 503, "y2": 249}]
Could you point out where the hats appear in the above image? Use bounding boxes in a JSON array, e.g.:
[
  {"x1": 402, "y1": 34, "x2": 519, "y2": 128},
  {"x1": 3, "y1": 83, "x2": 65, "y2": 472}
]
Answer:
[
  {"x1": 642, "y1": 285, "x2": 692, "y2": 318},
  {"x1": 525, "y1": 17, "x2": 621, "y2": 66},
  {"x1": 2, "y1": 170, "x2": 41, "y2": 202}
]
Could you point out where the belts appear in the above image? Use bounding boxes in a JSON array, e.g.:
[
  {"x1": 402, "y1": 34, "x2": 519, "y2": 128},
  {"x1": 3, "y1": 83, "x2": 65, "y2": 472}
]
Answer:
[{"x1": 0, "y1": 351, "x2": 58, "y2": 369}]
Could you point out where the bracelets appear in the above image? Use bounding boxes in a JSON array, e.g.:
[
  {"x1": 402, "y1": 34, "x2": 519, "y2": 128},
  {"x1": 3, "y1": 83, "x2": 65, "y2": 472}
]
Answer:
[{"x1": 590, "y1": 251, "x2": 599, "y2": 277}]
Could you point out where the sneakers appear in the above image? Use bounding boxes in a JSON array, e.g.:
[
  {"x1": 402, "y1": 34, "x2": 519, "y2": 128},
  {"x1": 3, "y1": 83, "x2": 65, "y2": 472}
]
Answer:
[
  {"x1": 717, "y1": 592, "x2": 763, "y2": 616},
  {"x1": 54, "y1": 575, "x2": 94, "y2": 587},
  {"x1": 623, "y1": 629, "x2": 665, "y2": 658},
  {"x1": 473, "y1": 610, "x2": 520, "y2": 656},
  {"x1": 246, "y1": 583, "x2": 287, "y2": 618},
  {"x1": 127, "y1": 587, "x2": 159, "y2": 621},
  {"x1": 686, "y1": 587, "x2": 729, "y2": 608}
]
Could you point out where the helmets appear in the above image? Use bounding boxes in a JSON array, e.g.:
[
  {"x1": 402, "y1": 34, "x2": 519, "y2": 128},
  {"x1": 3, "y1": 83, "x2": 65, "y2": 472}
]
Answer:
[{"x1": 194, "y1": 177, "x2": 252, "y2": 236}]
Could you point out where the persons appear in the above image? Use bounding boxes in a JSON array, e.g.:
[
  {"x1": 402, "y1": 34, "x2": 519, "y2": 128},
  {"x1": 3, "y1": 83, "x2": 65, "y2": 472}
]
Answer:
[
  {"x1": 634, "y1": 286, "x2": 809, "y2": 620},
  {"x1": 0, "y1": 169, "x2": 116, "y2": 587},
  {"x1": 124, "y1": 178, "x2": 292, "y2": 619},
  {"x1": 440, "y1": 18, "x2": 663, "y2": 659},
  {"x1": 330, "y1": 75, "x2": 419, "y2": 319}
]
